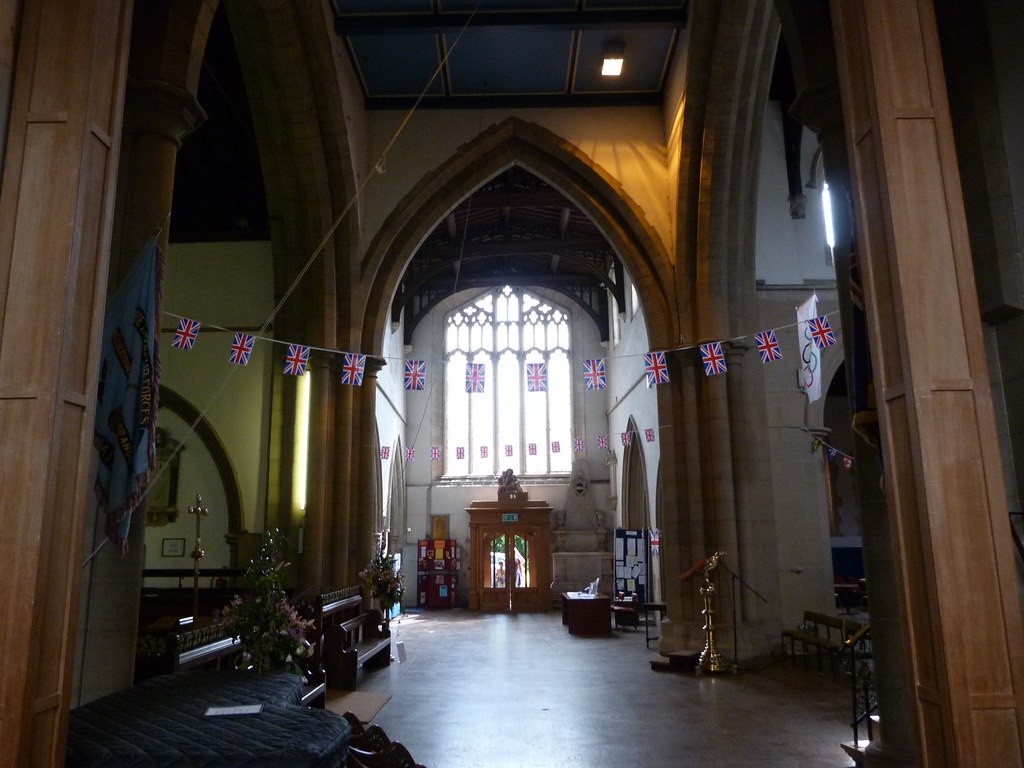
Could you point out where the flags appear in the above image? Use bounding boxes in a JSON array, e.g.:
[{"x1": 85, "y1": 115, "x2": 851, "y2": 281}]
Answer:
[
  {"x1": 95, "y1": 239, "x2": 163, "y2": 562},
  {"x1": 754, "y1": 330, "x2": 783, "y2": 363},
  {"x1": 621, "y1": 432, "x2": 631, "y2": 447},
  {"x1": 526, "y1": 363, "x2": 548, "y2": 391},
  {"x1": 644, "y1": 352, "x2": 669, "y2": 385},
  {"x1": 430, "y1": 447, "x2": 439, "y2": 461},
  {"x1": 582, "y1": 359, "x2": 606, "y2": 390},
  {"x1": 795, "y1": 294, "x2": 822, "y2": 403},
  {"x1": 380, "y1": 446, "x2": 390, "y2": 459},
  {"x1": 282, "y1": 344, "x2": 311, "y2": 376},
  {"x1": 456, "y1": 435, "x2": 607, "y2": 459},
  {"x1": 405, "y1": 447, "x2": 414, "y2": 461},
  {"x1": 700, "y1": 342, "x2": 726, "y2": 375},
  {"x1": 645, "y1": 429, "x2": 655, "y2": 442},
  {"x1": 813, "y1": 438, "x2": 852, "y2": 471},
  {"x1": 808, "y1": 316, "x2": 836, "y2": 348},
  {"x1": 229, "y1": 333, "x2": 255, "y2": 366},
  {"x1": 171, "y1": 319, "x2": 200, "y2": 351},
  {"x1": 341, "y1": 354, "x2": 366, "y2": 385},
  {"x1": 465, "y1": 363, "x2": 485, "y2": 393},
  {"x1": 404, "y1": 360, "x2": 426, "y2": 391}
]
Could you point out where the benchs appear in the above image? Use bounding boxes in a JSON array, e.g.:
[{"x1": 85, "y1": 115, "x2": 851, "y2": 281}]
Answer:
[
  {"x1": 782, "y1": 610, "x2": 869, "y2": 682},
  {"x1": 323, "y1": 608, "x2": 392, "y2": 692}
]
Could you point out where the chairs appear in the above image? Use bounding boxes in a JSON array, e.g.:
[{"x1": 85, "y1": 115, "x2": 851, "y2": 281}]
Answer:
[
  {"x1": 614, "y1": 592, "x2": 639, "y2": 632},
  {"x1": 610, "y1": 590, "x2": 625, "y2": 614}
]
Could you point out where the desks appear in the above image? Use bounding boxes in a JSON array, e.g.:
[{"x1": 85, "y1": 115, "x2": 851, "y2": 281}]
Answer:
[
  {"x1": 561, "y1": 592, "x2": 612, "y2": 636},
  {"x1": 642, "y1": 602, "x2": 666, "y2": 647},
  {"x1": 66, "y1": 666, "x2": 352, "y2": 768}
]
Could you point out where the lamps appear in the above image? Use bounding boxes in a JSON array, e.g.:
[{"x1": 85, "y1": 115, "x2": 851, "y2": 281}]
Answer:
[{"x1": 599, "y1": 37, "x2": 628, "y2": 78}]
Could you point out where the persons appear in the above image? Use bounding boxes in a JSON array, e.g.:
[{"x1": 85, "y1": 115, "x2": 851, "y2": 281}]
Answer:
[{"x1": 495, "y1": 550, "x2": 522, "y2": 588}]
